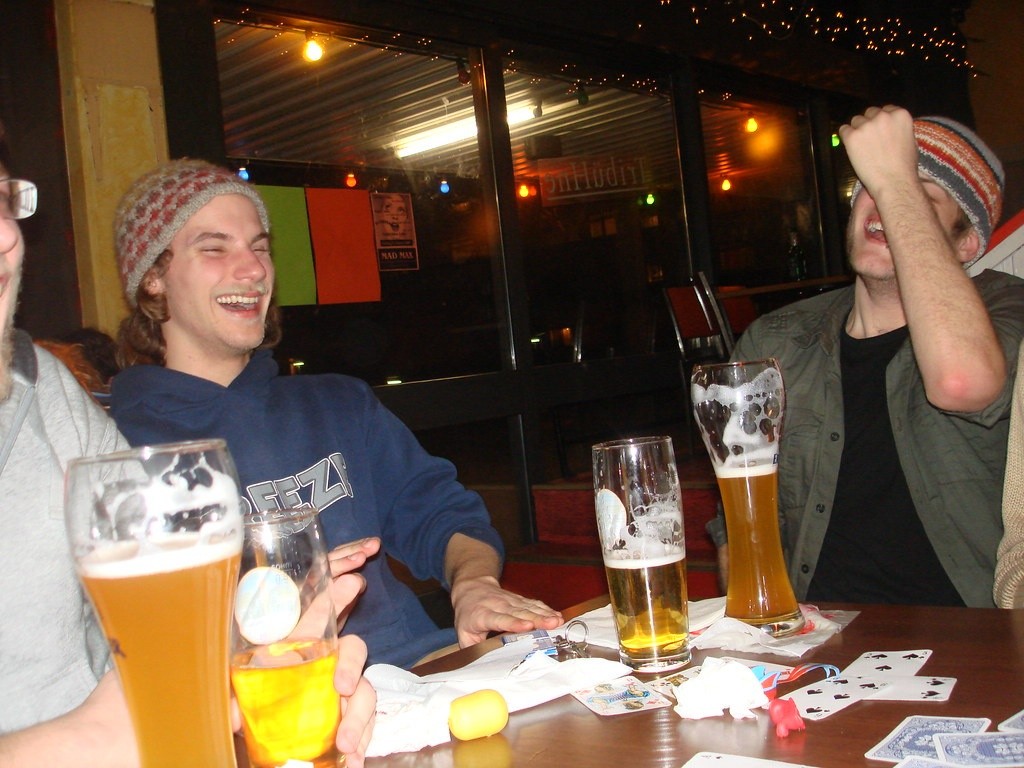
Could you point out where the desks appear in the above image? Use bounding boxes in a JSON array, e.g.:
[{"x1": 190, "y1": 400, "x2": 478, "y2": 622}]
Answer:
[
  {"x1": 713, "y1": 275, "x2": 849, "y2": 299},
  {"x1": 233, "y1": 593, "x2": 1024, "y2": 768}
]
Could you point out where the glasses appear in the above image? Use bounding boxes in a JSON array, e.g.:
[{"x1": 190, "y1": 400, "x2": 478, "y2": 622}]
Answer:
[{"x1": 0, "y1": 179, "x2": 38, "y2": 220}]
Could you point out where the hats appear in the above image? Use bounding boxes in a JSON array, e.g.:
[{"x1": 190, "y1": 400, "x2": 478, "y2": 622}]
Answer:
[
  {"x1": 850, "y1": 116, "x2": 1006, "y2": 272},
  {"x1": 112, "y1": 161, "x2": 269, "y2": 307}
]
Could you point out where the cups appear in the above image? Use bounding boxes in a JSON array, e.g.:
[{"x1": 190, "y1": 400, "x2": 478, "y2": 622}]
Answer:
[
  {"x1": 591, "y1": 435, "x2": 693, "y2": 673},
  {"x1": 63, "y1": 440, "x2": 246, "y2": 768},
  {"x1": 230, "y1": 507, "x2": 346, "y2": 768},
  {"x1": 690, "y1": 358, "x2": 807, "y2": 640}
]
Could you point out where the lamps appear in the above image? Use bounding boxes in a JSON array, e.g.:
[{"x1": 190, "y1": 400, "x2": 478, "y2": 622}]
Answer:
[
  {"x1": 574, "y1": 84, "x2": 589, "y2": 105},
  {"x1": 456, "y1": 59, "x2": 471, "y2": 85},
  {"x1": 303, "y1": 29, "x2": 324, "y2": 61}
]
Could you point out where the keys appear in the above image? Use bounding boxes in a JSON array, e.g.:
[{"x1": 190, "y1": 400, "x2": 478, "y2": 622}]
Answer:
[{"x1": 554, "y1": 621, "x2": 590, "y2": 660}]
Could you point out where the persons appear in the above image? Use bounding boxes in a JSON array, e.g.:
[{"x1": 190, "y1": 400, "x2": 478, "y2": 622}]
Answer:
[
  {"x1": 106, "y1": 159, "x2": 564, "y2": 648},
  {"x1": 0, "y1": 162, "x2": 380, "y2": 767},
  {"x1": 707, "y1": 103, "x2": 1024, "y2": 610}
]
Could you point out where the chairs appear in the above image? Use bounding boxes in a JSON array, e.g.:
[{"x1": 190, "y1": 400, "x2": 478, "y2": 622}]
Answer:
[{"x1": 661, "y1": 286, "x2": 759, "y2": 456}]
